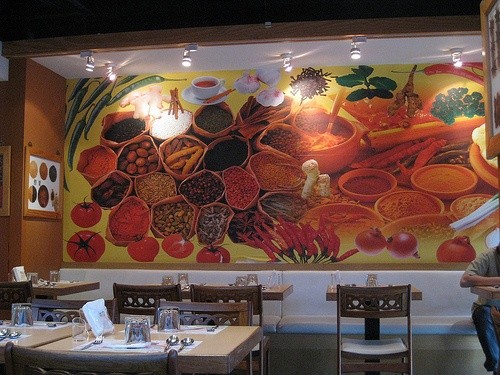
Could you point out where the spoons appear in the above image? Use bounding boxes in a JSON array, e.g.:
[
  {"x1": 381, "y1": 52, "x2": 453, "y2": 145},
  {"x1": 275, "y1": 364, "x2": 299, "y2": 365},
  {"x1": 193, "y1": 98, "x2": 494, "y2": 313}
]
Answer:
[
  {"x1": 164, "y1": 335, "x2": 179, "y2": 352},
  {"x1": 0, "y1": 329, "x2": 22, "y2": 341},
  {"x1": 178, "y1": 337, "x2": 194, "y2": 352}
]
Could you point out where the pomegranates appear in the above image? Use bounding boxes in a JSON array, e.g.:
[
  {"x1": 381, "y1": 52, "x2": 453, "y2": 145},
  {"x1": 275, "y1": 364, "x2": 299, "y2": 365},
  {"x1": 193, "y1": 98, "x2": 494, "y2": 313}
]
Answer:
[
  {"x1": 354, "y1": 226, "x2": 422, "y2": 259},
  {"x1": 435, "y1": 235, "x2": 476, "y2": 263}
]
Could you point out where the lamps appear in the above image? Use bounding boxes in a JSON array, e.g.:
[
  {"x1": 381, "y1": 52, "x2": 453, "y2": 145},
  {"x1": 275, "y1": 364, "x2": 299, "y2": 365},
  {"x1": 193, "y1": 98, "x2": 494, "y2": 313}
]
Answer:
[
  {"x1": 450, "y1": 48, "x2": 463, "y2": 68},
  {"x1": 182, "y1": 45, "x2": 199, "y2": 68},
  {"x1": 349, "y1": 37, "x2": 367, "y2": 60},
  {"x1": 105, "y1": 63, "x2": 118, "y2": 82},
  {"x1": 280, "y1": 52, "x2": 293, "y2": 73},
  {"x1": 80, "y1": 51, "x2": 95, "y2": 72}
]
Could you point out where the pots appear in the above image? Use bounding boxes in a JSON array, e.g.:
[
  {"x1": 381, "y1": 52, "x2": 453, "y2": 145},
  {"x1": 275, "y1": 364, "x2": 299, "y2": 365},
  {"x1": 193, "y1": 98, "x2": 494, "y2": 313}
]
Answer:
[{"x1": 297, "y1": 114, "x2": 369, "y2": 174}]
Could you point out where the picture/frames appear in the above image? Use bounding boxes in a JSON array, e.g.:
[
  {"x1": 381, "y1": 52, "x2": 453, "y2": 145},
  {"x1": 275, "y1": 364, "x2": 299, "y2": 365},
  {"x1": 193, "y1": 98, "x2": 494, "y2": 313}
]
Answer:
[
  {"x1": 0, "y1": 146, "x2": 11, "y2": 217},
  {"x1": 480, "y1": 0, "x2": 500, "y2": 160}
]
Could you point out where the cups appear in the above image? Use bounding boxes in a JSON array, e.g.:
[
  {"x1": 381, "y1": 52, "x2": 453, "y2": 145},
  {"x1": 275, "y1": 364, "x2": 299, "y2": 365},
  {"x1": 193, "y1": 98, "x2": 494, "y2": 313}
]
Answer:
[
  {"x1": 73, "y1": 317, "x2": 87, "y2": 343},
  {"x1": 192, "y1": 76, "x2": 225, "y2": 100}
]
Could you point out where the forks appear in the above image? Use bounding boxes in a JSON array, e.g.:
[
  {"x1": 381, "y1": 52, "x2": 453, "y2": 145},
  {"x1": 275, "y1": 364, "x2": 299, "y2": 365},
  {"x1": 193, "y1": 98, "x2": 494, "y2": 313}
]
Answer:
[{"x1": 81, "y1": 336, "x2": 104, "y2": 350}]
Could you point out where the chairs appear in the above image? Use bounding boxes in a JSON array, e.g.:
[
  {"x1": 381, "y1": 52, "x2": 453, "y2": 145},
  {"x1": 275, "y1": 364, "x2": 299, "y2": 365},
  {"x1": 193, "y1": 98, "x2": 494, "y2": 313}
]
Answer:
[{"x1": 0, "y1": 274, "x2": 413, "y2": 375}]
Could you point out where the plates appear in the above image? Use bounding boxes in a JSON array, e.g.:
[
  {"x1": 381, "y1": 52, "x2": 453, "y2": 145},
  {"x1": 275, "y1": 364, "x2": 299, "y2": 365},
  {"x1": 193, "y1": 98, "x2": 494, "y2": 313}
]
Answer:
[
  {"x1": 296, "y1": 204, "x2": 385, "y2": 243},
  {"x1": 182, "y1": 86, "x2": 230, "y2": 105},
  {"x1": 374, "y1": 191, "x2": 445, "y2": 222},
  {"x1": 379, "y1": 214, "x2": 467, "y2": 251},
  {"x1": 411, "y1": 164, "x2": 478, "y2": 200},
  {"x1": 450, "y1": 194, "x2": 494, "y2": 221},
  {"x1": 338, "y1": 168, "x2": 397, "y2": 203}
]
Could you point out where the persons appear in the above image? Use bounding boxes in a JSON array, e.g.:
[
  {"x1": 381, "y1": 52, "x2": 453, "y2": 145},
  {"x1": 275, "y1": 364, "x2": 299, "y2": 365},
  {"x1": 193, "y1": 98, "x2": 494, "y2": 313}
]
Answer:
[{"x1": 460, "y1": 242, "x2": 500, "y2": 375}]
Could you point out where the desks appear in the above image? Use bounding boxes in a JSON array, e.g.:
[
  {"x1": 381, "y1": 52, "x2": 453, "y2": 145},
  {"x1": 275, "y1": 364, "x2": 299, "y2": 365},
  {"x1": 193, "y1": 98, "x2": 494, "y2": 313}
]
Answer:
[
  {"x1": 36, "y1": 323, "x2": 262, "y2": 375},
  {"x1": 2, "y1": 281, "x2": 100, "y2": 299},
  {"x1": 326, "y1": 284, "x2": 422, "y2": 340},
  {"x1": 0, "y1": 320, "x2": 91, "y2": 375},
  {"x1": 470, "y1": 286, "x2": 500, "y2": 301},
  {"x1": 175, "y1": 283, "x2": 294, "y2": 301}
]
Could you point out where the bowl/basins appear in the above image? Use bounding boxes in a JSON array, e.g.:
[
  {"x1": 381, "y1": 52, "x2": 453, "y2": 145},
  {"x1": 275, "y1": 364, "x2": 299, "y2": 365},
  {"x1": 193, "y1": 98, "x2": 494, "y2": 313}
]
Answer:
[{"x1": 469, "y1": 143, "x2": 500, "y2": 191}]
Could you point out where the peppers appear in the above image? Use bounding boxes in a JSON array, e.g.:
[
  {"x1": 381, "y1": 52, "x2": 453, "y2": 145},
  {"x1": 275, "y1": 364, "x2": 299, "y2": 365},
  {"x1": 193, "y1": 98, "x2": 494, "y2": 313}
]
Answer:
[
  {"x1": 242, "y1": 216, "x2": 359, "y2": 265},
  {"x1": 64, "y1": 73, "x2": 187, "y2": 170}
]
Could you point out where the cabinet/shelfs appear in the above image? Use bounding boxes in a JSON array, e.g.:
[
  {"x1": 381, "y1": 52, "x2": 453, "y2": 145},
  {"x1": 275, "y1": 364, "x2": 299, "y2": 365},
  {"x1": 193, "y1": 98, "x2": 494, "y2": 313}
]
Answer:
[{"x1": 23, "y1": 146, "x2": 65, "y2": 221}]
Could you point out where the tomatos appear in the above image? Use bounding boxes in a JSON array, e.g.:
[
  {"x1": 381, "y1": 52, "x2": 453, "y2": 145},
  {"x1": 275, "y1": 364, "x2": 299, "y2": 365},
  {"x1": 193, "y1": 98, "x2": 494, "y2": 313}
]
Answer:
[
  {"x1": 127, "y1": 234, "x2": 231, "y2": 264},
  {"x1": 64, "y1": 195, "x2": 105, "y2": 262}
]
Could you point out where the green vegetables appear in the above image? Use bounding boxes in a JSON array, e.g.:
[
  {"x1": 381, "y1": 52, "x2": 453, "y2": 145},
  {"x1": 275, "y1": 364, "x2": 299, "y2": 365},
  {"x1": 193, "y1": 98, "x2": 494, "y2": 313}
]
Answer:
[{"x1": 431, "y1": 87, "x2": 487, "y2": 125}]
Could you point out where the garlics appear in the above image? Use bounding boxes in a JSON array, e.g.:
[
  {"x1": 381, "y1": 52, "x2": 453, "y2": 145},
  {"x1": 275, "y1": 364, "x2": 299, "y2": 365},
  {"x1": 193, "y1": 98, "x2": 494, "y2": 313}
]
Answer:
[{"x1": 234, "y1": 67, "x2": 285, "y2": 107}]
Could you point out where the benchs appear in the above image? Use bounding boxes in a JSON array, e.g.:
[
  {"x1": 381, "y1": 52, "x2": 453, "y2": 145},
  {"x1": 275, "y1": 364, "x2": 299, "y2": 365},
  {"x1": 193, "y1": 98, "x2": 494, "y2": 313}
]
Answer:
[{"x1": 55, "y1": 261, "x2": 482, "y2": 375}]
